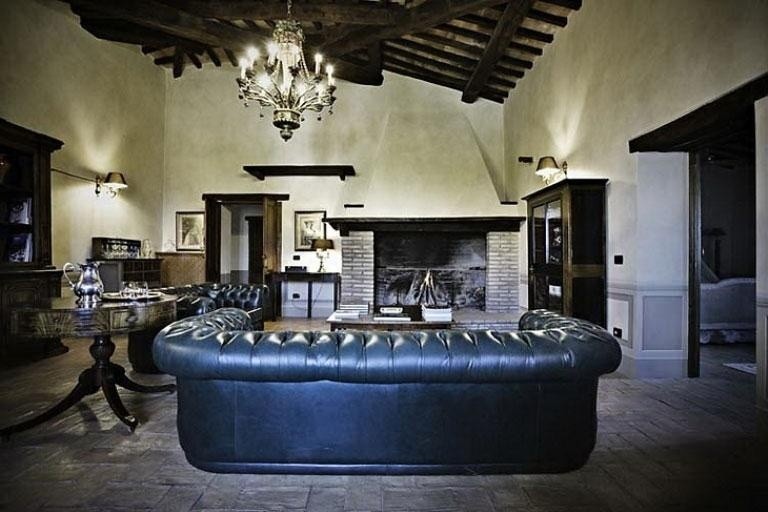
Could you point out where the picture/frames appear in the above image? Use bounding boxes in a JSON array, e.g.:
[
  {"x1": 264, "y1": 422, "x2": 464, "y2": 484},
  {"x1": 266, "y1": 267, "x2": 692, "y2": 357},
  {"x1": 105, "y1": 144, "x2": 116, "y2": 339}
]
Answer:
[
  {"x1": 175, "y1": 211, "x2": 207, "y2": 252},
  {"x1": 293, "y1": 210, "x2": 326, "y2": 252}
]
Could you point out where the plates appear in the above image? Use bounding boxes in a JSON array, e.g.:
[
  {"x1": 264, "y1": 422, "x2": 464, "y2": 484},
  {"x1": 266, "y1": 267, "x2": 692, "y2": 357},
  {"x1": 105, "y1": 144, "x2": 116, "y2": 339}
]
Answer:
[{"x1": 102, "y1": 288, "x2": 163, "y2": 300}]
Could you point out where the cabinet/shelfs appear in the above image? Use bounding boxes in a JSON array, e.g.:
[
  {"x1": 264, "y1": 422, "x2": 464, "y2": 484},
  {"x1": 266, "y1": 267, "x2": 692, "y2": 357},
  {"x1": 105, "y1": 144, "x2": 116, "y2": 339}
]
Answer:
[
  {"x1": 0, "y1": 116, "x2": 71, "y2": 367},
  {"x1": 88, "y1": 257, "x2": 165, "y2": 296},
  {"x1": 279, "y1": 271, "x2": 339, "y2": 320},
  {"x1": 520, "y1": 178, "x2": 608, "y2": 332}
]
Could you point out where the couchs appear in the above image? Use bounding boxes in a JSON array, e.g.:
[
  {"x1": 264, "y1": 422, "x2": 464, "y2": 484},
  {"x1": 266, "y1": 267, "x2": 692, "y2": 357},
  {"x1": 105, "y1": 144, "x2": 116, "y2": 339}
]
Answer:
[
  {"x1": 128, "y1": 283, "x2": 269, "y2": 373},
  {"x1": 152, "y1": 308, "x2": 622, "y2": 475}
]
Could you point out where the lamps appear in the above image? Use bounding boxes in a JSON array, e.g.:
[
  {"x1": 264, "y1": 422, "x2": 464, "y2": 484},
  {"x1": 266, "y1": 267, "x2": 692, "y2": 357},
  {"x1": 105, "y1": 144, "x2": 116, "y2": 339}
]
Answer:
[
  {"x1": 236, "y1": 2, "x2": 336, "y2": 141},
  {"x1": 534, "y1": 156, "x2": 566, "y2": 187},
  {"x1": 94, "y1": 168, "x2": 128, "y2": 199},
  {"x1": 310, "y1": 239, "x2": 335, "y2": 273}
]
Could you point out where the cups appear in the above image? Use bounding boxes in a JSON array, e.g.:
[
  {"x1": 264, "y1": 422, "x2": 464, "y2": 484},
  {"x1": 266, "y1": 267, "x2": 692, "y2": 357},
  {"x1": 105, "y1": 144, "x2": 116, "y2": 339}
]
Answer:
[
  {"x1": 133, "y1": 280, "x2": 149, "y2": 299},
  {"x1": 119, "y1": 280, "x2": 136, "y2": 300}
]
